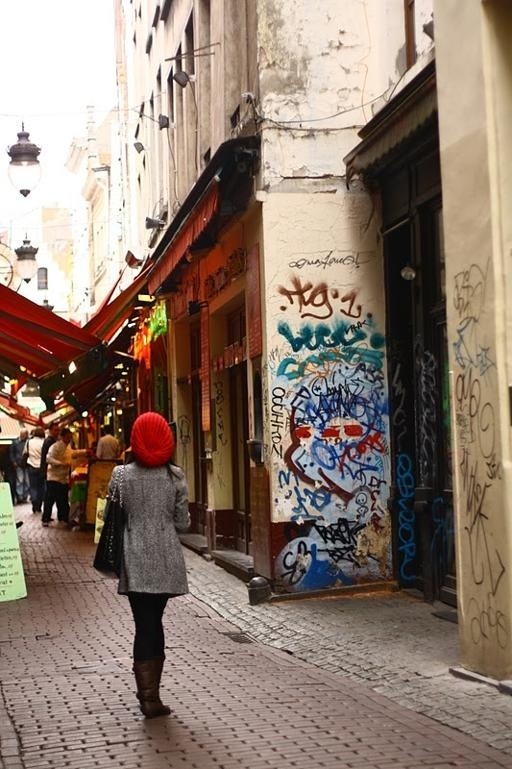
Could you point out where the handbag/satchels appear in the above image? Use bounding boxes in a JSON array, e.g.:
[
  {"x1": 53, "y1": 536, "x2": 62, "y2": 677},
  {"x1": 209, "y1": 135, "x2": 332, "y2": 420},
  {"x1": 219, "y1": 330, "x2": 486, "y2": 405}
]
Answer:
[
  {"x1": 91, "y1": 466, "x2": 128, "y2": 580},
  {"x1": 18, "y1": 439, "x2": 30, "y2": 471}
]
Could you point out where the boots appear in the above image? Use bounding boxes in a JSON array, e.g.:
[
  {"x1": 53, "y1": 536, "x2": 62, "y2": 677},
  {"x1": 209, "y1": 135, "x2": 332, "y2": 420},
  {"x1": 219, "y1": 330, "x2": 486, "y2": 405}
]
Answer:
[
  {"x1": 132, "y1": 656, "x2": 171, "y2": 719},
  {"x1": 31, "y1": 497, "x2": 42, "y2": 513}
]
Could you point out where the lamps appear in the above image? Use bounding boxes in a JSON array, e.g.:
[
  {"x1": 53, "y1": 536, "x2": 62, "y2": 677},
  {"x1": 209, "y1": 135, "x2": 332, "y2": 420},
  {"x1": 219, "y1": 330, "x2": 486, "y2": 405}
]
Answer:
[
  {"x1": 133, "y1": 142, "x2": 144, "y2": 154},
  {"x1": 172, "y1": 71, "x2": 194, "y2": 98},
  {"x1": 67, "y1": 344, "x2": 106, "y2": 374},
  {"x1": 6, "y1": 117, "x2": 45, "y2": 286},
  {"x1": 399, "y1": 260, "x2": 424, "y2": 284},
  {"x1": 186, "y1": 299, "x2": 207, "y2": 317},
  {"x1": 136, "y1": 285, "x2": 156, "y2": 303},
  {"x1": 157, "y1": 113, "x2": 170, "y2": 130}
]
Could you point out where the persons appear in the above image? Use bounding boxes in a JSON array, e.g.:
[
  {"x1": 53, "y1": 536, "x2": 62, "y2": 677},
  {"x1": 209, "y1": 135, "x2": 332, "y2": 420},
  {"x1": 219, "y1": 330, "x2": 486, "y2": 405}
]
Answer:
[
  {"x1": 109, "y1": 412, "x2": 191, "y2": 716},
  {"x1": 11, "y1": 423, "x2": 119, "y2": 527}
]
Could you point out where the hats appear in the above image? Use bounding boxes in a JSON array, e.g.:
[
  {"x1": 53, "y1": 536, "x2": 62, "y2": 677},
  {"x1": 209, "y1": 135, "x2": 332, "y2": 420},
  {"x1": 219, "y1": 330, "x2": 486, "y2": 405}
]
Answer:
[{"x1": 129, "y1": 410, "x2": 176, "y2": 469}]
